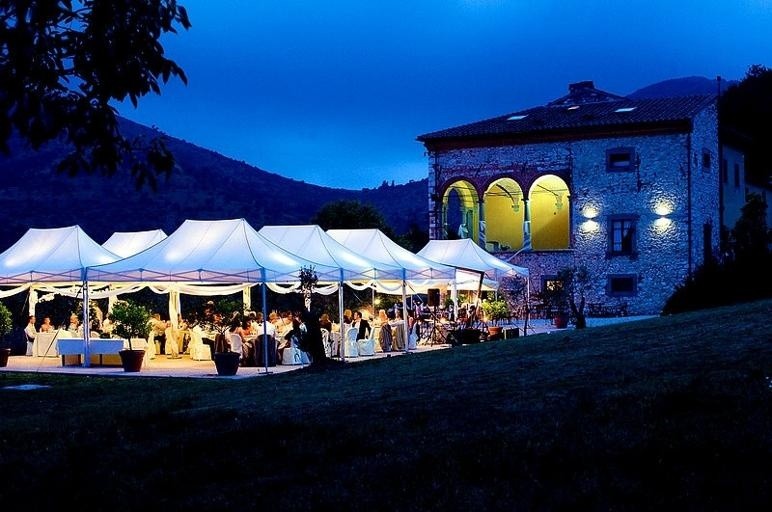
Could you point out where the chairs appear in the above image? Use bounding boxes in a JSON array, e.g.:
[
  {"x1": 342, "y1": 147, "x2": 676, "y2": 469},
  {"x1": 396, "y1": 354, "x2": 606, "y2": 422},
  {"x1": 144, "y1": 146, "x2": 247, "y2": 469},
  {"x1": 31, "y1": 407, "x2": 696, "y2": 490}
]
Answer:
[
  {"x1": 162, "y1": 324, "x2": 420, "y2": 367},
  {"x1": 24, "y1": 324, "x2": 158, "y2": 366}
]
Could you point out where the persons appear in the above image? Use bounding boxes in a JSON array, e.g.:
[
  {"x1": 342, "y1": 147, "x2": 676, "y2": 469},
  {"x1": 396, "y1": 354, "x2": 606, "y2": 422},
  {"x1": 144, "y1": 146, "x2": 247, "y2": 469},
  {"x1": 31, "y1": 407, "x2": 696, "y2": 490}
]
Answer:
[{"x1": 26, "y1": 305, "x2": 479, "y2": 367}]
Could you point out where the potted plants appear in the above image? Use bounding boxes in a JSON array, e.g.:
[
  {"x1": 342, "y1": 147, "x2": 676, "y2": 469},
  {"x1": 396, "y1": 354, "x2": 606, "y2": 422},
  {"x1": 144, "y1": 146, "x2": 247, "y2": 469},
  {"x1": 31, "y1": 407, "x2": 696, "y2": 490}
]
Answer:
[
  {"x1": 481, "y1": 301, "x2": 509, "y2": 335},
  {"x1": 107, "y1": 299, "x2": 154, "y2": 372},
  {"x1": 0, "y1": 301, "x2": 13, "y2": 367},
  {"x1": 547, "y1": 287, "x2": 570, "y2": 329},
  {"x1": 186, "y1": 298, "x2": 255, "y2": 376}
]
